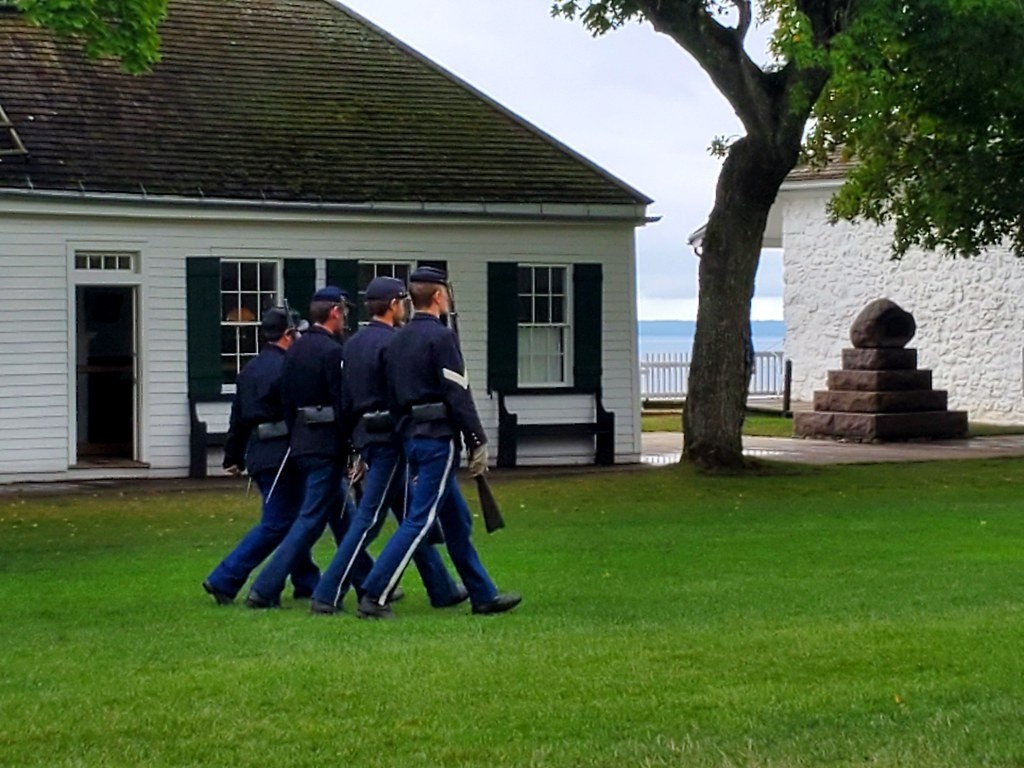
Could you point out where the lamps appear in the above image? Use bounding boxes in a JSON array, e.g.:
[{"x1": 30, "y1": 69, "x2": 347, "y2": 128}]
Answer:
[{"x1": 226, "y1": 307, "x2": 256, "y2": 321}]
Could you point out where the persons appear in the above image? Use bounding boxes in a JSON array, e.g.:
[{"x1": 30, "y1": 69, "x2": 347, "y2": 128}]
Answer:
[
  {"x1": 201, "y1": 307, "x2": 322, "y2": 607},
  {"x1": 358, "y1": 272, "x2": 522, "y2": 619},
  {"x1": 244, "y1": 289, "x2": 378, "y2": 610},
  {"x1": 309, "y1": 278, "x2": 469, "y2": 615},
  {"x1": 85, "y1": 321, "x2": 133, "y2": 444}
]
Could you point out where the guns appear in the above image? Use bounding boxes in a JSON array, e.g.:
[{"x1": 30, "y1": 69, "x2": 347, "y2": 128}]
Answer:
[
  {"x1": 282, "y1": 299, "x2": 304, "y2": 342},
  {"x1": 449, "y1": 281, "x2": 507, "y2": 536},
  {"x1": 341, "y1": 292, "x2": 364, "y2": 507},
  {"x1": 404, "y1": 279, "x2": 448, "y2": 545}
]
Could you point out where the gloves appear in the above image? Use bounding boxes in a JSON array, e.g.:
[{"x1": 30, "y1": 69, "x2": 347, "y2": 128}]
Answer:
[{"x1": 470, "y1": 444, "x2": 490, "y2": 478}]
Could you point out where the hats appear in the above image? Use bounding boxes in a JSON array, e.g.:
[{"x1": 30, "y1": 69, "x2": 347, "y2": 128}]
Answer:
[
  {"x1": 262, "y1": 310, "x2": 301, "y2": 332},
  {"x1": 365, "y1": 277, "x2": 408, "y2": 301},
  {"x1": 409, "y1": 266, "x2": 448, "y2": 285},
  {"x1": 314, "y1": 286, "x2": 348, "y2": 303}
]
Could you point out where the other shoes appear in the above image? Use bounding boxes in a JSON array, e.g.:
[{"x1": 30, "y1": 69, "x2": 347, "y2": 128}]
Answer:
[
  {"x1": 245, "y1": 590, "x2": 280, "y2": 609},
  {"x1": 203, "y1": 580, "x2": 233, "y2": 606},
  {"x1": 471, "y1": 594, "x2": 522, "y2": 616},
  {"x1": 293, "y1": 587, "x2": 312, "y2": 598},
  {"x1": 431, "y1": 585, "x2": 469, "y2": 608},
  {"x1": 356, "y1": 599, "x2": 392, "y2": 618},
  {"x1": 310, "y1": 600, "x2": 336, "y2": 613},
  {"x1": 390, "y1": 590, "x2": 404, "y2": 601}
]
